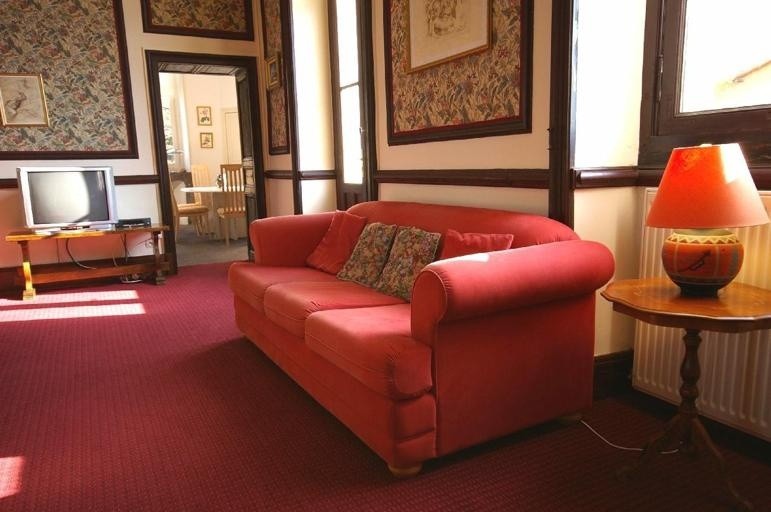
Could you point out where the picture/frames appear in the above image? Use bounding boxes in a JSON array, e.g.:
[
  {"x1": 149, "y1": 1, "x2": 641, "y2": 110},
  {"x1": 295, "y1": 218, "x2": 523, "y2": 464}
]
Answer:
[
  {"x1": 200, "y1": 132, "x2": 213, "y2": 148},
  {"x1": 265, "y1": 53, "x2": 282, "y2": 90},
  {"x1": 197, "y1": 106, "x2": 211, "y2": 126},
  {"x1": 0, "y1": 73, "x2": 50, "y2": 127},
  {"x1": 405, "y1": 1, "x2": 493, "y2": 73}
]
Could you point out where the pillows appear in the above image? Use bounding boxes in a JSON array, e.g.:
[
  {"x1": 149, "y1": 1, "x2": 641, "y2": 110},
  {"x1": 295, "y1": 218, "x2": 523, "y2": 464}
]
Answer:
[
  {"x1": 337, "y1": 222, "x2": 394, "y2": 287},
  {"x1": 373, "y1": 226, "x2": 439, "y2": 302},
  {"x1": 439, "y1": 229, "x2": 514, "y2": 261},
  {"x1": 308, "y1": 211, "x2": 367, "y2": 275}
]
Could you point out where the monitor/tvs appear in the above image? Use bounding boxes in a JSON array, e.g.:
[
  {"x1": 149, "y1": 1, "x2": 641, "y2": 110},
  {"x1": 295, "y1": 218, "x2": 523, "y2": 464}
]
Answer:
[{"x1": 15, "y1": 165, "x2": 119, "y2": 235}]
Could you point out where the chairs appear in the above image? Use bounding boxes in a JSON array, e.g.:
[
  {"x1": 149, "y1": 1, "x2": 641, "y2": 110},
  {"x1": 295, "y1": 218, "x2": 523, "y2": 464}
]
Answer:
[
  {"x1": 219, "y1": 164, "x2": 248, "y2": 243},
  {"x1": 169, "y1": 168, "x2": 211, "y2": 244}
]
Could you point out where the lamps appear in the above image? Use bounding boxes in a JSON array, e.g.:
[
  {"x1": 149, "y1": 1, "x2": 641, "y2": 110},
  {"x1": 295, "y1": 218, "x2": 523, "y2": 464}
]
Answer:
[{"x1": 645, "y1": 143, "x2": 769, "y2": 299}]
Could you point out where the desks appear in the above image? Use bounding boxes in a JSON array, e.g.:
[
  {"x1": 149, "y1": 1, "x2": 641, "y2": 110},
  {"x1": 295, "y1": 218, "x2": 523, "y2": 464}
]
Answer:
[
  {"x1": 5, "y1": 224, "x2": 169, "y2": 300},
  {"x1": 600, "y1": 276, "x2": 771, "y2": 512}
]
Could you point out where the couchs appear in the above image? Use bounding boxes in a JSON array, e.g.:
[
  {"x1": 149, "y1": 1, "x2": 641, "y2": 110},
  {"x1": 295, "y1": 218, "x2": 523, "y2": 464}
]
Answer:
[{"x1": 228, "y1": 201, "x2": 613, "y2": 478}]
